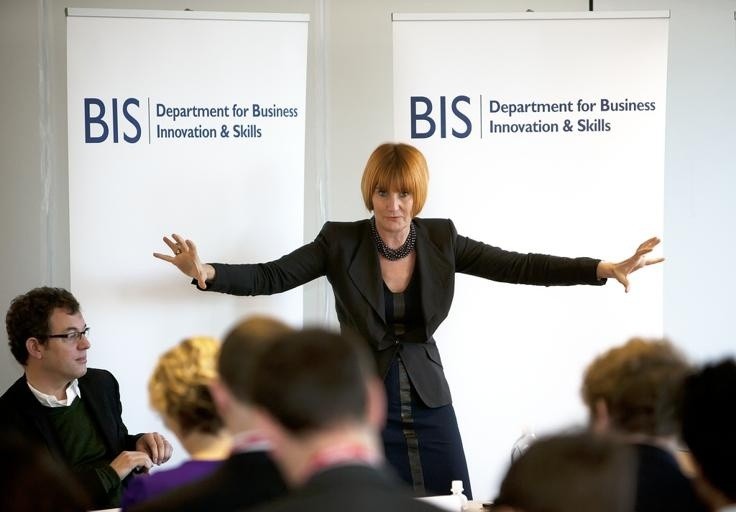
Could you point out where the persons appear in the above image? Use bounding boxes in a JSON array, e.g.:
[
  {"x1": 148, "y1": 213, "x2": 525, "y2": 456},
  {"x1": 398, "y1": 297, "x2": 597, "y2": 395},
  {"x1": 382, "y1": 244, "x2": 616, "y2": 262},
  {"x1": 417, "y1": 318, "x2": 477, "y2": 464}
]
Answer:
[
  {"x1": 152, "y1": 143, "x2": 665, "y2": 499},
  {"x1": 122, "y1": 335, "x2": 230, "y2": 507},
  {"x1": 250, "y1": 326, "x2": 443, "y2": 511},
  {"x1": 135, "y1": 317, "x2": 297, "y2": 511},
  {"x1": 493, "y1": 337, "x2": 736, "y2": 512},
  {"x1": 0, "y1": 287, "x2": 173, "y2": 510}
]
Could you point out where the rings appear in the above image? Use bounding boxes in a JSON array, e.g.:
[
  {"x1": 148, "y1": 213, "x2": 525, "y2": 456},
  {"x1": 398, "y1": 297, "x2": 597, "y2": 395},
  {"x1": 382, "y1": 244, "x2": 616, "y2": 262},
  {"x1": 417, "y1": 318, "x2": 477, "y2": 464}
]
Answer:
[{"x1": 175, "y1": 249, "x2": 181, "y2": 255}]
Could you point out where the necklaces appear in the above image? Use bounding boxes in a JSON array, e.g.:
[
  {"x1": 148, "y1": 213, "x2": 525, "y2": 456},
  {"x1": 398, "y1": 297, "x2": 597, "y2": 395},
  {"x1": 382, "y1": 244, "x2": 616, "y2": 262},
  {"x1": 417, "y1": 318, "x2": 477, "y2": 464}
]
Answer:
[{"x1": 370, "y1": 219, "x2": 416, "y2": 260}]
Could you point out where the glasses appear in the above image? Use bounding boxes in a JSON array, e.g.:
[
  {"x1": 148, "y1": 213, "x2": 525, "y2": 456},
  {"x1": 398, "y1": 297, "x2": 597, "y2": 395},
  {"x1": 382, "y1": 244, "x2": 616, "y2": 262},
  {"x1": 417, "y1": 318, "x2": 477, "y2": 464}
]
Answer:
[{"x1": 48, "y1": 327, "x2": 89, "y2": 342}]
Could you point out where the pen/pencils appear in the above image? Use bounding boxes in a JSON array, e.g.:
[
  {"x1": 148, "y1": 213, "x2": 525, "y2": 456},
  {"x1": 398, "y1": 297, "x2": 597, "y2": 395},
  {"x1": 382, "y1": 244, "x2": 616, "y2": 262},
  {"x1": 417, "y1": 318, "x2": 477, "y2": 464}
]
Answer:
[{"x1": 135, "y1": 456, "x2": 153, "y2": 474}]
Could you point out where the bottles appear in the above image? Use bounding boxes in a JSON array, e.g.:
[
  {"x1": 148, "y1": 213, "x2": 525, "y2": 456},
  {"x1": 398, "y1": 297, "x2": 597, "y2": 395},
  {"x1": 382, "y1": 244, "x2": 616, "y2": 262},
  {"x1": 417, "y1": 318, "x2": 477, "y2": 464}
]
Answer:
[{"x1": 450, "y1": 480, "x2": 468, "y2": 512}]
